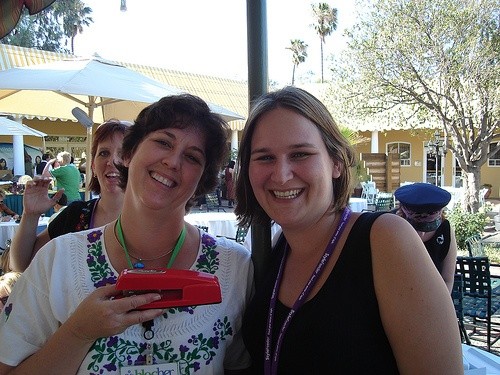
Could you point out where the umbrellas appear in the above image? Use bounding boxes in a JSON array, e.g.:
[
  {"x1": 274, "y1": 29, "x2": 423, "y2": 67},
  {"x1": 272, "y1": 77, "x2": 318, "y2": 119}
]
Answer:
[
  {"x1": 0, "y1": 117, "x2": 47, "y2": 137},
  {"x1": 0, "y1": 51, "x2": 246, "y2": 124}
]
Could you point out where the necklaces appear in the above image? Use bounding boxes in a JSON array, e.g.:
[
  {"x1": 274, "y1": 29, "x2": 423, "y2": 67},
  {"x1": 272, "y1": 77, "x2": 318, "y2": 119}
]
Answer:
[{"x1": 113, "y1": 218, "x2": 175, "y2": 268}]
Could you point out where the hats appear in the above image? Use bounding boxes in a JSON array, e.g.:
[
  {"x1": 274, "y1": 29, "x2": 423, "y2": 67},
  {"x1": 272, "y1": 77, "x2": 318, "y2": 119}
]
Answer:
[{"x1": 393, "y1": 183, "x2": 451, "y2": 232}]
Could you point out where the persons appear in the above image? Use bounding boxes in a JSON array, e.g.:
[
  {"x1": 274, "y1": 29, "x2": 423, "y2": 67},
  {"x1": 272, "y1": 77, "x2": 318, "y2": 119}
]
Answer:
[
  {"x1": 234, "y1": 87, "x2": 464, "y2": 375},
  {"x1": 36, "y1": 154, "x2": 51, "y2": 175},
  {"x1": 35, "y1": 156, "x2": 41, "y2": 164},
  {"x1": 225, "y1": 161, "x2": 235, "y2": 208},
  {"x1": 9, "y1": 119, "x2": 128, "y2": 272},
  {"x1": 0, "y1": 158, "x2": 13, "y2": 181},
  {"x1": 47, "y1": 192, "x2": 68, "y2": 227},
  {"x1": 42, "y1": 151, "x2": 81, "y2": 206},
  {"x1": 390, "y1": 183, "x2": 457, "y2": 295},
  {"x1": 0, "y1": 94, "x2": 253, "y2": 375}
]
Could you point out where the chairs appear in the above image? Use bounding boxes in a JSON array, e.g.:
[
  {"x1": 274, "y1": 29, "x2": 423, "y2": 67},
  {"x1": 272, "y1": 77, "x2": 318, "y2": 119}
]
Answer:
[
  {"x1": 451, "y1": 256, "x2": 500, "y2": 353},
  {"x1": 217, "y1": 223, "x2": 250, "y2": 245},
  {"x1": 205, "y1": 191, "x2": 234, "y2": 212},
  {"x1": 358, "y1": 181, "x2": 396, "y2": 214}
]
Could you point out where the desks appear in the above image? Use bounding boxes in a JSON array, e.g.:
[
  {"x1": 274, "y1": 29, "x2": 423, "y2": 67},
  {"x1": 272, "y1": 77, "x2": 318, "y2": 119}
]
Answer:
[
  {"x1": 349, "y1": 197, "x2": 368, "y2": 212},
  {"x1": 1, "y1": 190, "x2": 85, "y2": 219},
  {"x1": 0, "y1": 219, "x2": 49, "y2": 255},
  {"x1": 183, "y1": 213, "x2": 280, "y2": 254}
]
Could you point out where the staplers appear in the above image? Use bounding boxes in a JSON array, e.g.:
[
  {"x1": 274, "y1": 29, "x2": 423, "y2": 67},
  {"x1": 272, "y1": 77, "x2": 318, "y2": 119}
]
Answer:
[{"x1": 113, "y1": 266, "x2": 223, "y2": 310}]
